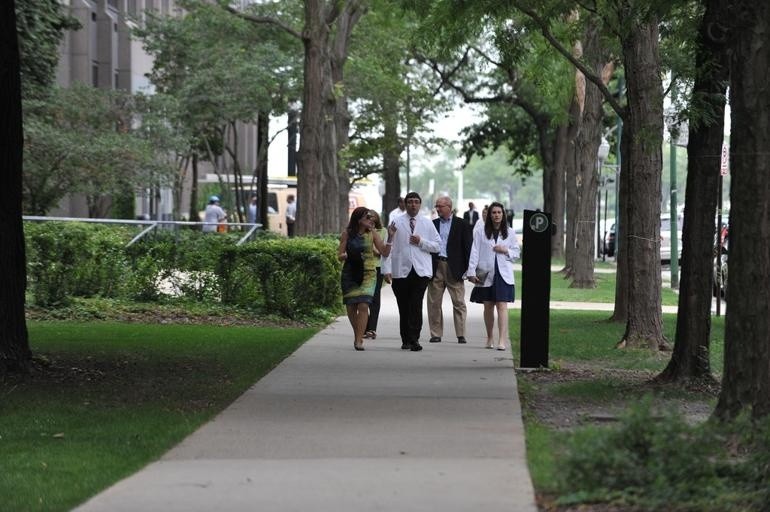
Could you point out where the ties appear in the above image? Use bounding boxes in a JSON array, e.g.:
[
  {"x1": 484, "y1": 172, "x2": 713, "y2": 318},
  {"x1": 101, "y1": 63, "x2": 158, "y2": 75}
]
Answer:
[{"x1": 409, "y1": 218, "x2": 414, "y2": 233}]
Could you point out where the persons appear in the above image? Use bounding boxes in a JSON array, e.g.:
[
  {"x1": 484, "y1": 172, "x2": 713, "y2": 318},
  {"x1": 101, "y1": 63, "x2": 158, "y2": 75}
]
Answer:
[
  {"x1": 380, "y1": 192, "x2": 443, "y2": 350},
  {"x1": 427, "y1": 197, "x2": 473, "y2": 343},
  {"x1": 467, "y1": 202, "x2": 521, "y2": 350},
  {"x1": 362, "y1": 209, "x2": 388, "y2": 338},
  {"x1": 337, "y1": 207, "x2": 397, "y2": 350},
  {"x1": 388, "y1": 197, "x2": 516, "y2": 230},
  {"x1": 285, "y1": 194, "x2": 297, "y2": 237},
  {"x1": 248, "y1": 194, "x2": 258, "y2": 224},
  {"x1": 202, "y1": 196, "x2": 227, "y2": 233}
]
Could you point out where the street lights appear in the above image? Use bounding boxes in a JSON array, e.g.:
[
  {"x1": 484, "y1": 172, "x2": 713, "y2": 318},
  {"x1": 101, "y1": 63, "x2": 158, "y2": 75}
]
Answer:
[
  {"x1": 596, "y1": 137, "x2": 610, "y2": 260},
  {"x1": 664, "y1": 105, "x2": 681, "y2": 289}
]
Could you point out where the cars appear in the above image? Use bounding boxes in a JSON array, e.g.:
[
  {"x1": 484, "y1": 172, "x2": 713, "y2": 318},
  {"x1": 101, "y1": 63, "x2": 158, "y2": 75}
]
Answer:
[{"x1": 594, "y1": 211, "x2": 729, "y2": 305}]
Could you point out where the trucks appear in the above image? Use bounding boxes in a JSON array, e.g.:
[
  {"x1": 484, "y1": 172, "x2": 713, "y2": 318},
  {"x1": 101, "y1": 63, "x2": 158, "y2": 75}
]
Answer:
[{"x1": 198, "y1": 184, "x2": 366, "y2": 238}]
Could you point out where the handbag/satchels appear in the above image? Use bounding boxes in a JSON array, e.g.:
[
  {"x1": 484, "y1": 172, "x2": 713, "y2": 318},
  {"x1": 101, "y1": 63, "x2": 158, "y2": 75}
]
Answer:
[{"x1": 461, "y1": 267, "x2": 488, "y2": 284}]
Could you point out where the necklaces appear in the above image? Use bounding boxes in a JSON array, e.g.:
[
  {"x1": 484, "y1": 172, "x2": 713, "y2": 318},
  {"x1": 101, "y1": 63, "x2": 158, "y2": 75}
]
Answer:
[{"x1": 357, "y1": 232, "x2": 365, "y2": 236}]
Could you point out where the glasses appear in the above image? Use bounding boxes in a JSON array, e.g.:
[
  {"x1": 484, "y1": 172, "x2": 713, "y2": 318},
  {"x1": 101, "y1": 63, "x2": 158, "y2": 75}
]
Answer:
[
  {"x1": 361, "y1": 214, "x2": 373, "y2": 220},
  {"x1": 434, "y1": 204, "x2": 447, "y2": 208}
]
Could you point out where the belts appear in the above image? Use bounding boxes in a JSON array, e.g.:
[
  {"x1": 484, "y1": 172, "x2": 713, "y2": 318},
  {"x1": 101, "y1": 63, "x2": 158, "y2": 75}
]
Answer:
[{"x1": 437, "y1": 255, "x2": 447, "y2": 262}]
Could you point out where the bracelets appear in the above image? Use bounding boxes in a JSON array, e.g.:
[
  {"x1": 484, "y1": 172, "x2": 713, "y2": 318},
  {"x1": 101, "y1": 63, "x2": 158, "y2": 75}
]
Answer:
[{"x1": 386, "y1": 241, "x2": 394, "y2": 246}]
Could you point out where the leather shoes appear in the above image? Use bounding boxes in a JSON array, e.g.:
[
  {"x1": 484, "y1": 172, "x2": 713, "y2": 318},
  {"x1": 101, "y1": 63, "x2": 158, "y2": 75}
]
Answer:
[
  {"x1": 354, "y1": 330, "x2": 376, "y2": 351},
  {"x1": 486, "y1": 344, "x2": 493, "y2": 348},
  {"x1": 497, "y1": 345, "x2": 505, "y2": 350},
  {"x1": 410, "y1": 343, "x2": 422, "y2": 351},
  {"x1": 430, "y1": 337, "x2": 441, "y2": 342},
  {"x1": 401, "y1": 344, "x2": 409, "y2": 349},
  {"x1": 458, "y1": 336, "x2": 466, "y2": 343}
]
